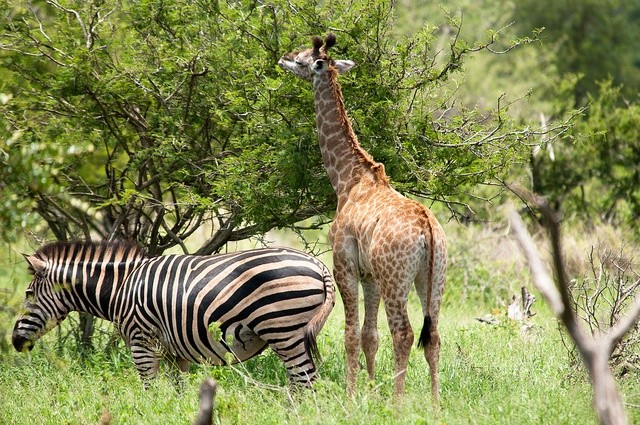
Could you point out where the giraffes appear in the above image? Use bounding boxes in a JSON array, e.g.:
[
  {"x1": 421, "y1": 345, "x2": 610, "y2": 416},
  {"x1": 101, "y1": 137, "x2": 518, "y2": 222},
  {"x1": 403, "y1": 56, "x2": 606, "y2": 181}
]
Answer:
[{"x1": 279, "y1": 34, "x2": 449, "y2": 414}]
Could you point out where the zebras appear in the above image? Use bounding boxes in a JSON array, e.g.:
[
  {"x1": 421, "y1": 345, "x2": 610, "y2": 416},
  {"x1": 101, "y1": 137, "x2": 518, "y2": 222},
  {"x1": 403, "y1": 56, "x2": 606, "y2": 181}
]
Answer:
[{"x1": 13, "y1": 244, "x2": 335, "y2": 397}]
